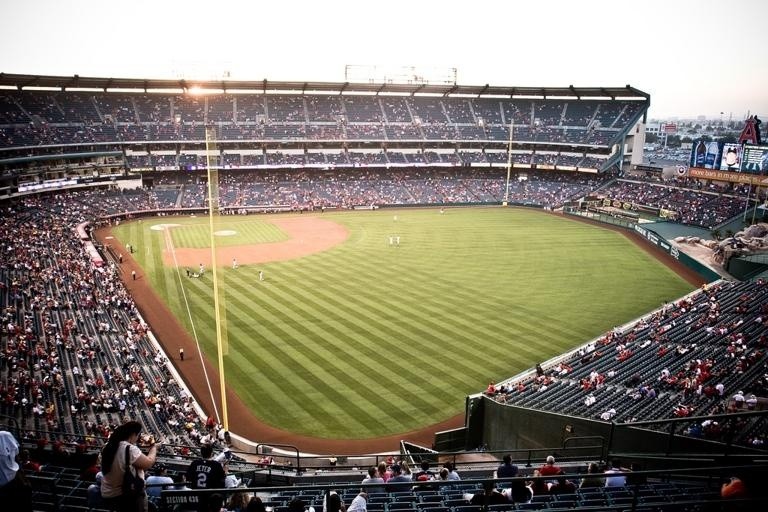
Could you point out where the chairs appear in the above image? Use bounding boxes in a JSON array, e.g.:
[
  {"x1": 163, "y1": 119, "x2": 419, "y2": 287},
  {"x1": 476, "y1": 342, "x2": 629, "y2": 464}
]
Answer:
[
  {"x1": 0, "y1": 91, "x2": 644, "y2": 226},
  {"x1": 227, "y1": 278, "x2": 767, "y2": 510},
  {"x1": 0, "y1": 231, "x2": 228, "y2": 512}
]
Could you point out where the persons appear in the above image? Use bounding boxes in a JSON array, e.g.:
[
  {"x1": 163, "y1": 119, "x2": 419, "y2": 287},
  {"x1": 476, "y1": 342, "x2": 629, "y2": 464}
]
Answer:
[
  {"x1": 507, "y1": 273, "x2": 768, "y2": 450},
  {"x1": 259, "y1": 270, "x2": 264, "y2": 281},
  {"x1": 2, "y1": 212, "x2": 206, "y2": 511},
  {"x1": 0, "y1": 89, "x2": 644, "y2": 216},
  {"x1": 388, "y1": 235, "x2": 401, "y2": 249},
  {"x1": 201, "y1": 408, "x2": 633, "y2": 512},
  {"x1": 576, "y1": 167, "x2": 768, "y2": 231},
  {"x1": 720, "y1": 475, "x2": 743, "y2": 497},
  {"x1": 232, "y1": 258, "x2": 239, "y2": 270}
]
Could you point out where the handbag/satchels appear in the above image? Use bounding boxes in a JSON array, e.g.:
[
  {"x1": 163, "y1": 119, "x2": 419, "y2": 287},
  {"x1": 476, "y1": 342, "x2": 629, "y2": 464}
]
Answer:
[{"x1": 123, "y1": 465, "x2": 145, "y2": 497}]
